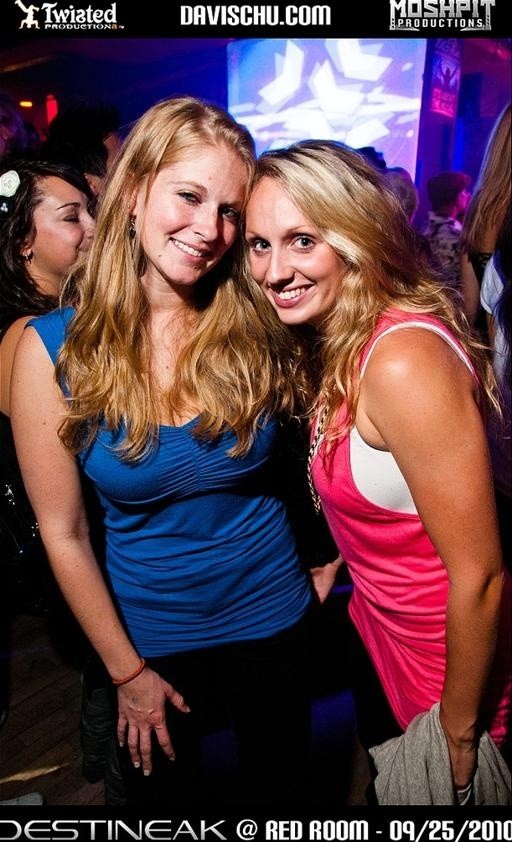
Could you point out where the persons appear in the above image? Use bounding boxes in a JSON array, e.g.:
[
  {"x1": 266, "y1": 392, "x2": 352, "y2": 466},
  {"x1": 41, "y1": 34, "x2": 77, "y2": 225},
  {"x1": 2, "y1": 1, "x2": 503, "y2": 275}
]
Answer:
[
  {"x1": 0, "y1": 159, "x2": 117, "y2": 784},
  {"x1": 244, "y1": 141, "x2": 512, "y2": 806},
  {"x1": 8, "y1": 97, "x2": 325, "y2": 805},
  {"x1": 460, "y1": 103, "x2": 512, "y2": 326},
  {"x1": 420, "y1": 171, "x2": 471, "y2": 289},
  {"x1": 478, "y1": 202, "x2": 511, "y2": 392}
]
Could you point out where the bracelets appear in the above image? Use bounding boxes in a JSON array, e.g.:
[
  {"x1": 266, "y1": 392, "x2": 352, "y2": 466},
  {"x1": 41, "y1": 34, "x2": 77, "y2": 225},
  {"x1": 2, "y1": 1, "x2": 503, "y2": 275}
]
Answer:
[{"x1": 113, "y1": 658, "x2": 145, "y2": 685}]
